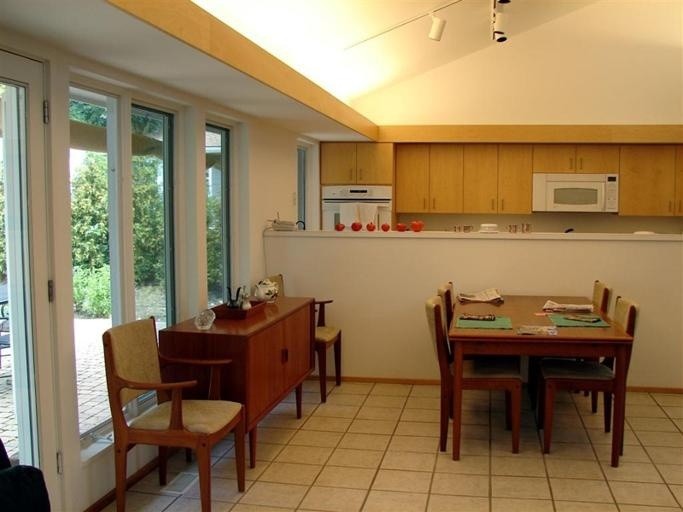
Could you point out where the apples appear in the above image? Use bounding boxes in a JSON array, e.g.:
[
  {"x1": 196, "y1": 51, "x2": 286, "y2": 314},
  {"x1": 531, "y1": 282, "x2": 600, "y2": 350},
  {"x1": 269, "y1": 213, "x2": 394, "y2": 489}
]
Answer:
[
  {"x1": 350, "y1": 221, "x2": 363, "y2": 232},
  {"x1": 335, "y1": 222, "x2": 345, "y2": 231},
  {"x1": 410, "y1": 220, "x2": 424, "y2": 233},
  {"x1": 366, "y1": 221, "x2": 377, "y2": 232},
  {"x1": 396, "y1": 223, "x2": 407, "y2": 232},
  {"x1": 381, "y1": 223, "x2": 390, "y2": 232}
]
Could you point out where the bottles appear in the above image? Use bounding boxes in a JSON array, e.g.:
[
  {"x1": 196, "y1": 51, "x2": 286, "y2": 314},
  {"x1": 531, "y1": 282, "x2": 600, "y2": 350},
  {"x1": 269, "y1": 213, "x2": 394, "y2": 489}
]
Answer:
[
  {"x1": 242, "y1": 292, "x2": 251, "y2": 310},
  {"x1": 191, "y1": 308, "x2": 216, "y2": 329}
]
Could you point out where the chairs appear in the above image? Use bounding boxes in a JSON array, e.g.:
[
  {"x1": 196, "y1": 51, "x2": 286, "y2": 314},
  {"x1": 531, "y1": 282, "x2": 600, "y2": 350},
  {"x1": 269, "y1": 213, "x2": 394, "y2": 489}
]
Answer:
[
  {"x1": 426, "y1": 280, "x2": 638, "y2": 468},
  {"x1": 102, "y1": 317, "x2": 245, "y2": 511},
  {"x1": 260, "y1": 274, "x2": 341, "y2": 403}
]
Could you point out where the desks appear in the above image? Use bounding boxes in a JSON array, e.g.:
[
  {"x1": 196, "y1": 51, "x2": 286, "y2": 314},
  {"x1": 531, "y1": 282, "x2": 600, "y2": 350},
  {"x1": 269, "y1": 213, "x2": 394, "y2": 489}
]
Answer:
[{"x1": 159, "y1": 296, "x2": 315, "y2": 468}]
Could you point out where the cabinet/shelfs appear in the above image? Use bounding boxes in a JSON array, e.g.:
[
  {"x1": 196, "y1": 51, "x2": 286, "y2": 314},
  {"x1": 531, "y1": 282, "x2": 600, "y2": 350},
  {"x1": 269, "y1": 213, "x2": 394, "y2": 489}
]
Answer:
[
  {"x1": 532, "y1": 144, "x2": 620, "y2": 174},
  {"x1": 320, "y1": 143, "x2": 395, "y2": 185},
  {"x1": 464, "y1": 143, "x2": 532, "y2": 214},
  {"x1": 395, "y1": 144, "x2": 464, "y2": 214},
  {"x1": 619, "y1": 145, "x2": 683, "y2": 217}
]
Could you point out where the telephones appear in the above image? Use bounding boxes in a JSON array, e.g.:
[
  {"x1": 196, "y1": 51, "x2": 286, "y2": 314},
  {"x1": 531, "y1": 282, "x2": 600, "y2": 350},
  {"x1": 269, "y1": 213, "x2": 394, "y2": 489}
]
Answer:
[{"x1": 272, "y1": 218, "x2": 296, "y2": 231}]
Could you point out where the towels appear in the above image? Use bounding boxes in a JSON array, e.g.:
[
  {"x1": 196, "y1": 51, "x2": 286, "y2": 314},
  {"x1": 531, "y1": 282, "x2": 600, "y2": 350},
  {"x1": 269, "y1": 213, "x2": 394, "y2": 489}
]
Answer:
[{"x1": 340, "y1": 202, "x2": 377, "y2": 228}]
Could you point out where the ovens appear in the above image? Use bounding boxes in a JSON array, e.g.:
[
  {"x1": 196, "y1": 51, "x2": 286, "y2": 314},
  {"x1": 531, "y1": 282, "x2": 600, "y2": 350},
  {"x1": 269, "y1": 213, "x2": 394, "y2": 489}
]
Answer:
[{"x1": 321, "y1": 200, "x2": 392, "y2": 232}]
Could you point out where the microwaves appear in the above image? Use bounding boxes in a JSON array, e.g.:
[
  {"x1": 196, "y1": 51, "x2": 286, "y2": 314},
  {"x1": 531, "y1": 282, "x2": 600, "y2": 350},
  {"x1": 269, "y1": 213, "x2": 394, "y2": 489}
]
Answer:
[{"x1": 532, "y1": 173, "x2": 620, "y2": 213}]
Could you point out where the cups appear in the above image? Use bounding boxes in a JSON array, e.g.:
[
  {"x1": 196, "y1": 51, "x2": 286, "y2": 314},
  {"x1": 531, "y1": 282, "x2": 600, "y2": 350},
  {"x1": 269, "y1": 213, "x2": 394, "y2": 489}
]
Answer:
[
  {"x1": 502, "y1": 223, "x2": 517, "y2": 234},
  {"x1": 521, "y1": 223, "x2": 535, "y2": 234},
  {"x1": 462, "y1": 225, "x2": 473, "y2": 234}
]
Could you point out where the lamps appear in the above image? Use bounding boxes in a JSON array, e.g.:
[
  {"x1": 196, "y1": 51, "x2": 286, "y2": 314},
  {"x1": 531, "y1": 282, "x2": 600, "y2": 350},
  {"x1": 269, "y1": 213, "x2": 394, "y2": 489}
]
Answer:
[{"x1": 345, "y1": 0, "x2": 511, "y2": 49}]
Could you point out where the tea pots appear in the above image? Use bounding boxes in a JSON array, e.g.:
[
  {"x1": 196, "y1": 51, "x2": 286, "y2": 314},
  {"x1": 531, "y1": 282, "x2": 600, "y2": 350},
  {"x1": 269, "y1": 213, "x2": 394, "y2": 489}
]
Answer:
[{"x1": 253, "y1": 278, "x2": 280, "y2": 304}]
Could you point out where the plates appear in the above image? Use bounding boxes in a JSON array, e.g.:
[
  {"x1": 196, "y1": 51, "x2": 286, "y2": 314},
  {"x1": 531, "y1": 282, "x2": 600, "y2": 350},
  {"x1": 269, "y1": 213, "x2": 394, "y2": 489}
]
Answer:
[{"x1": 478, "y1": 230, "x2": 499, "y2": 233}]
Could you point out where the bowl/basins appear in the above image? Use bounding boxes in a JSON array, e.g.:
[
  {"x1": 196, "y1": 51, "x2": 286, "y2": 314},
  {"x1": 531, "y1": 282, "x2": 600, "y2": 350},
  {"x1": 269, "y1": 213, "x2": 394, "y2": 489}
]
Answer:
[{"x1": 480, "y1": 223, "x2": 498, "y2": 232}]
